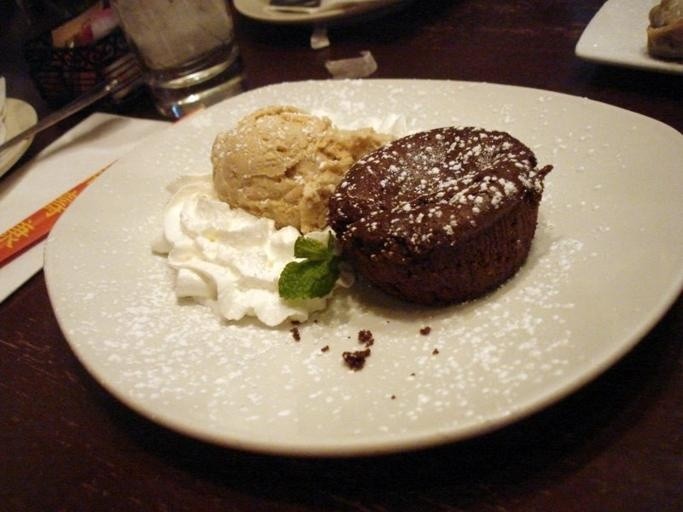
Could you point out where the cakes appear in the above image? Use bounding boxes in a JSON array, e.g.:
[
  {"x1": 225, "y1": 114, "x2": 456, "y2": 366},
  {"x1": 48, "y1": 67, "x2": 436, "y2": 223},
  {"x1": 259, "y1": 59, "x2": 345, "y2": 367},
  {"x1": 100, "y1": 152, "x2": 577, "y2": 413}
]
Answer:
[{"x1": 326, "y1": 124, "x2": 553, "y2": 307}]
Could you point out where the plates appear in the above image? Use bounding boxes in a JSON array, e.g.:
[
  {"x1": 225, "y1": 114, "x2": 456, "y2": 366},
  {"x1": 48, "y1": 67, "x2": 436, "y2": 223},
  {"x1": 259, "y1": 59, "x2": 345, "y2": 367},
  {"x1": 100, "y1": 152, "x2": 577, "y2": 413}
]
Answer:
[
  {"x1": 42, "y1": 76, "x2": 682, "y2": 457},
  {"x1": 573, "y1": 0, "x2": 681, "y2": 73}
]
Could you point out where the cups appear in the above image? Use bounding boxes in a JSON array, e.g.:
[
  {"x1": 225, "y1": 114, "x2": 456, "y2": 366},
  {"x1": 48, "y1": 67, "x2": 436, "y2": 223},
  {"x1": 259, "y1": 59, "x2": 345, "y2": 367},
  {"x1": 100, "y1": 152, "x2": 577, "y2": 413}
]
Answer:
[{"x1": 109, "y1": 2, "x2": 247, "y2": 121}]
